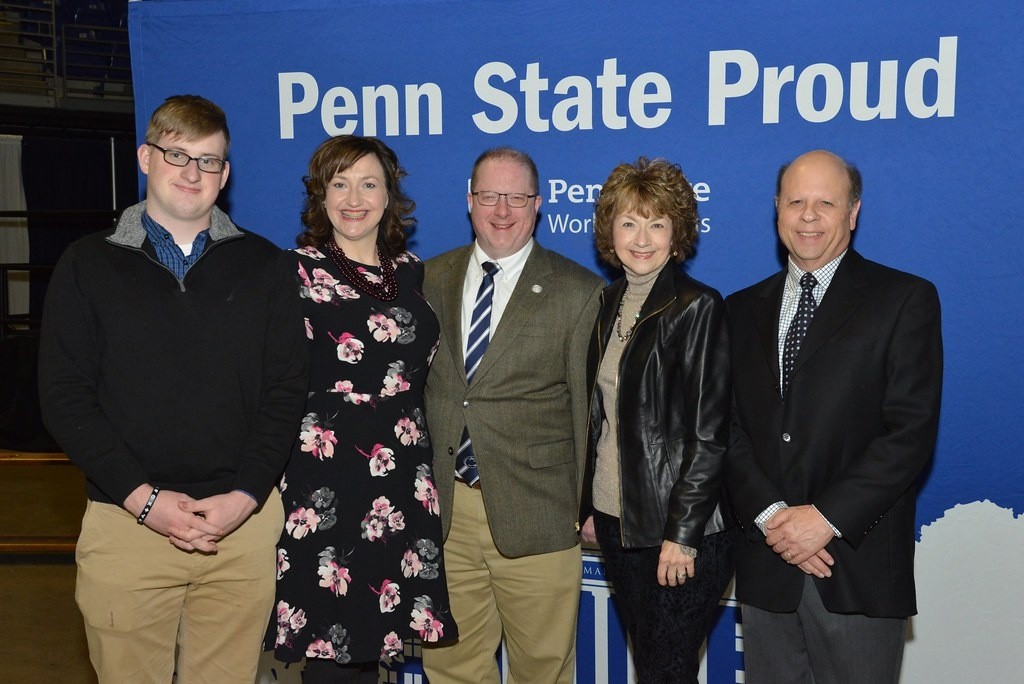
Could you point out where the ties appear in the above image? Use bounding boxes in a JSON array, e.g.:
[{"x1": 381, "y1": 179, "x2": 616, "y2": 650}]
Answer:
[
  {"x1": 782, "y1": 271, "x2": 819, "y2": 401},
  {"x1": 455, "y1": 262, "x2": 500, "y2": 487}
]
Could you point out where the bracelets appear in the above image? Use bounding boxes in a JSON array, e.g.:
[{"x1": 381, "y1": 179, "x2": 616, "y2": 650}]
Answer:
[{"x1": 137, "y1": 487, "x2": 159, "y2": 525}]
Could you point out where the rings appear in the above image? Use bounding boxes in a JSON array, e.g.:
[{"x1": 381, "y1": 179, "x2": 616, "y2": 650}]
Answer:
[
  {"x1": 677, "y1": 573, "x2": 687, "y2": 576},
  {"x1": 784, "y1": 549, "x2": 793, "y2": 560}
]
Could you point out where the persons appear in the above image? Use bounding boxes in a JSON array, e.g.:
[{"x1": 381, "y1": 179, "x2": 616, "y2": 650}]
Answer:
[
  {"x1": 575, "y1": 156, "x2": 735, "y2": 683},
  {"x1": 262, "y1": 134, "x2": 461, "y2": 684},
  {"x1": 726, "y1": 150, "x2": 943, "y2": 684},
  {"x1": 420, "y1": 146, "x2": 604, "y2": 684},
  {"x1": 35, "y1": 94, "x2": 305, "y2": 683}
]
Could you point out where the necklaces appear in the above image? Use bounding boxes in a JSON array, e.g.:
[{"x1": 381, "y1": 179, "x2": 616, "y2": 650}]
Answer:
[
  {"x1": 326, "y1": 234, "x2": 399, "y2": 301},
  {"x1": 617, "y1": 284, "x2": 643, "y2": 342}
]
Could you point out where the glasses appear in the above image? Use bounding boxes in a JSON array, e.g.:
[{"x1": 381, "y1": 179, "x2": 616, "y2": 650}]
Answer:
[
  {"x1": 147, "y1": 142, "x2": 226, "y2": 173},
  {"x1": 472, "y1": 189, "x2": 538, "y2": 208}
]
